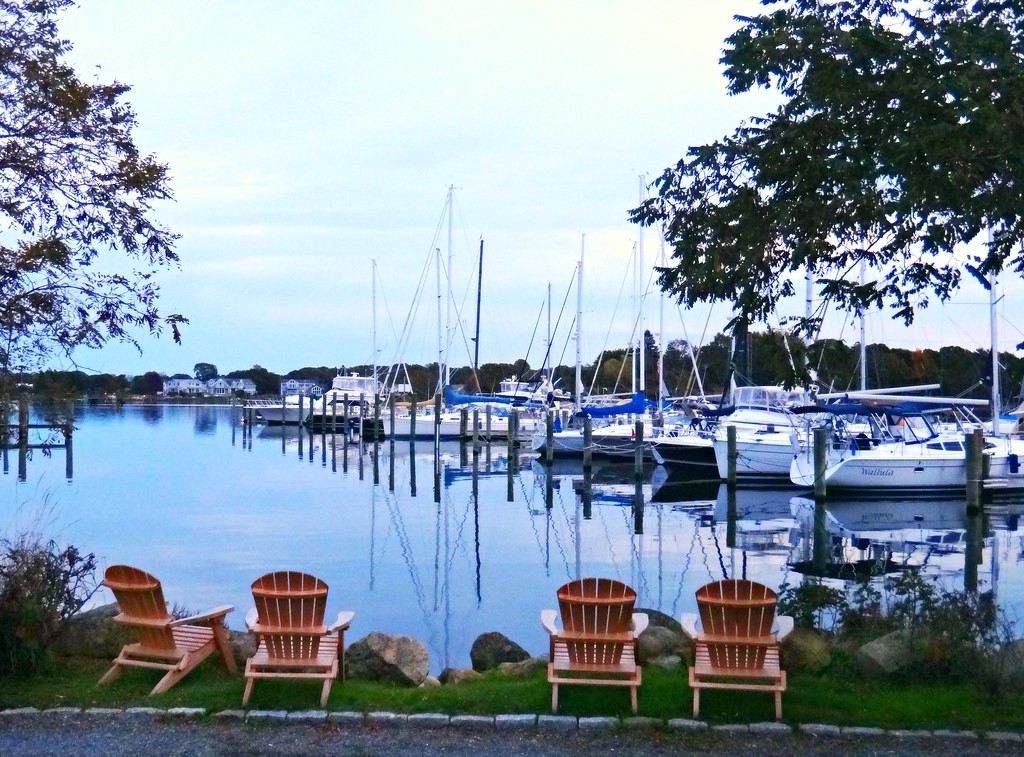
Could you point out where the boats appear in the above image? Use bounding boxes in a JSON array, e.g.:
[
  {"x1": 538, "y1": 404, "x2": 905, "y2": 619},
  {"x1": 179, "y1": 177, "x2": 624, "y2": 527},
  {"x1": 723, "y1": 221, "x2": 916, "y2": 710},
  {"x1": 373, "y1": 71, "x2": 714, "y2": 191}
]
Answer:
[{"x1": 241, "y1": 382, "x2": 318, "y2": 424}]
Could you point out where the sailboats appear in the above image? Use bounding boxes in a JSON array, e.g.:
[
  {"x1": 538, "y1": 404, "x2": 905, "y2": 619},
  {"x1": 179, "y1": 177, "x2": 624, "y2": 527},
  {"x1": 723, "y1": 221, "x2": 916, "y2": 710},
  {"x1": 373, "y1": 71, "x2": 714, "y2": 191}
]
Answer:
[
  {"x1": 301, "y1": 186, "x2": 1024, "y2": 492},
  {"x1": 298, "y1": 428, "x2": 1023, "y2": 617}
]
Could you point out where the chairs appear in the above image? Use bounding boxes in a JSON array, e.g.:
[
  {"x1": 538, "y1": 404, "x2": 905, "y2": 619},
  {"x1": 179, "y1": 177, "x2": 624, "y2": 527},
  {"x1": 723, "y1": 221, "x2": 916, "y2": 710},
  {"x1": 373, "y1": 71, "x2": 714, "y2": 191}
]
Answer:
[
  {"x1": 241, "y1": 569, "x2": 358, "y2": 708},
  {"x1": 90, "y1": 565, "x2": 237, "y2": 697},
  {"x1": 678, "y1": 579, "x2": 788, "y2": 723},
  {"x1": 539, "y1": 577, "x2": 651, "y2": 714}
]
